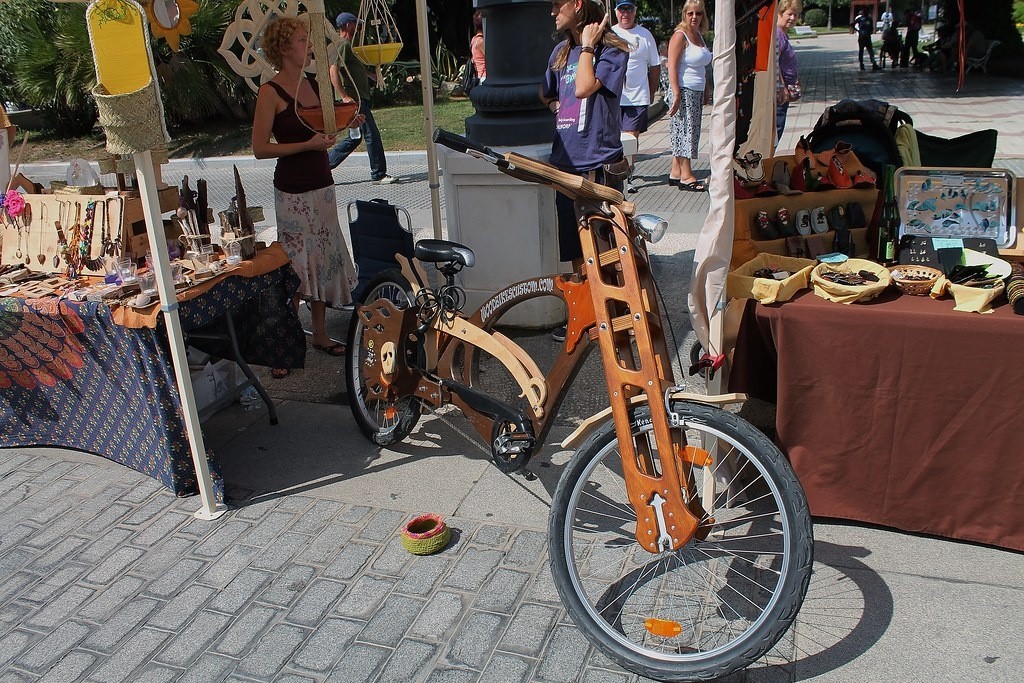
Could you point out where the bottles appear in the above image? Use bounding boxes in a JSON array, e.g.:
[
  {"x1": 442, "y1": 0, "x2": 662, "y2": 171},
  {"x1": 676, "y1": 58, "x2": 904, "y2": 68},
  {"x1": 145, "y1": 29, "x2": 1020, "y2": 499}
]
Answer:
[{"x1": 867, "y1": 164, "x2": 900, "y2": 269}]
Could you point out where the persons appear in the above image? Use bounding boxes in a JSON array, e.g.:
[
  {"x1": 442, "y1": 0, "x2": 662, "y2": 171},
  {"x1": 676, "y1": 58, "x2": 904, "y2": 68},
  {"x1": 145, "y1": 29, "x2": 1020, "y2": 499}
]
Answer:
[
  {"x1": 943, "y1": 20, "x2": 987, "y2": 79},
  {"x1": 773, "y1": 0, "x2": 802, "y2": 156},
  {"x1": 855, "y1": 11, "x2": 863, "y2": 41},
  {"x1": 849, "y1": 8, "x2": 878, "y2": 70},
  {"x1": 610, "y1": 0, "x2": 661, "y2": 192},
  {"x1": 251, "y1": 19, "x2": 358, "y2": 378},
  {"x1": 902, "y1": 9, "x2": 921, "y2": 67},
  {"x1": 666, "y1": 0, "x2": 711, "y2": 190},
  {"x1": 327, "y1": 13, "x2": 400, "y2": 185},
  {"x1": 539, "y1": 0, "x2": 634, "y2": 341},
  {"x1": 923, "y1": 25, "x2": 950, "y2": 66},
  {"x1": 880, "y1": 21, "x2": 902, "y2": 67},
  {"x1": 658, "y1": 41, "x2": 669, "y2": 89},
  {"x1": 880, "y1": 9, "x2": 893, "y2": 28},
  {"x1": 470, "y1": 10, "x2": 486, "y2": 86}
]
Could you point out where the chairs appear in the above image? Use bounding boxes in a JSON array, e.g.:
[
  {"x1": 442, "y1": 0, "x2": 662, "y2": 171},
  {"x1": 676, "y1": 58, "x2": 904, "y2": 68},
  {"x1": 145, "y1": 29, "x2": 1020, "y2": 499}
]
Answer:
[{"x1": 302, "y1": 198, "x2": 414, "y2": 346}]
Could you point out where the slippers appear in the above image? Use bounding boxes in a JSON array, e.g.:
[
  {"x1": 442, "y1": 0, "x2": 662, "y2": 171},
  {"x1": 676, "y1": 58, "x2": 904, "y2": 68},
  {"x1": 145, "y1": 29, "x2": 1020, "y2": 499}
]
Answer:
[
  {"x1": 678, "y1": 180, "x2": 705, "y2": 192},
  {"x1": 668, "y1": 178, "x2": 680, "y2": 186},
  {"x1": 312, "y1": 341, "x2": 347, "y2": 356},
  {"x1": 271, "y1": 367, "x2": 291, "y2": 379}
]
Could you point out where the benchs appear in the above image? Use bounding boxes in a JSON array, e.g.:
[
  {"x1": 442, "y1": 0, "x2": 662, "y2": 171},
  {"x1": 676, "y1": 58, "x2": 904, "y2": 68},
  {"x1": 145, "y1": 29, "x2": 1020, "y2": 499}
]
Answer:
[
  {"x1": 793, "y1": 26, "x2": 817, "y2": 35},
  {"x1": 965, "y1": 40, "x2": 1001, "y2": 74}
]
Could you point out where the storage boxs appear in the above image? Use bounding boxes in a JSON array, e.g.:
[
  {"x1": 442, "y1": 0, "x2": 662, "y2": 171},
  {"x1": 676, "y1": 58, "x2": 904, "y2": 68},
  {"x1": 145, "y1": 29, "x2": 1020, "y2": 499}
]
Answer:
[{"x1": 103, "y1": 186, "x2": 182, "y2": 262}]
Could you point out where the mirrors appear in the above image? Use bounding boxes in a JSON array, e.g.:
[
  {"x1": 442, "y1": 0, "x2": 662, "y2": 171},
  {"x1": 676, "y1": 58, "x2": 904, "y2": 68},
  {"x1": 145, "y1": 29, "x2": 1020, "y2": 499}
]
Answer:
[{"x1": 143, "y1": 0, "x2": 199, "y2": 53}]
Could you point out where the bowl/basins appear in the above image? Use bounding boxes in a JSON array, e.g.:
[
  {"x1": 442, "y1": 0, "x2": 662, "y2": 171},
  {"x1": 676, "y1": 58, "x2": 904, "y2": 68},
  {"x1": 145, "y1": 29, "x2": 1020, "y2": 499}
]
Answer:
[
  {"x1": 353, "y1": 43, "x2": 402, "y2": 65},
  {"x1": 298, "y1": 102, "x2": 359, "y2": 130}
]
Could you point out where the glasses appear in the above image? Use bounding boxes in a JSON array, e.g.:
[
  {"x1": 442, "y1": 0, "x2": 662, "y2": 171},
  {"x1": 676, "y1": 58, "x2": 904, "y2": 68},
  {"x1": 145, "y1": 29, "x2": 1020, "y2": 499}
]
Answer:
[
  {"x1": 686, "y1": 11, "x2": 702, "y2": 16},
  {"x1": 618, "y1": 6, "x2": 634, "y2": 13}
]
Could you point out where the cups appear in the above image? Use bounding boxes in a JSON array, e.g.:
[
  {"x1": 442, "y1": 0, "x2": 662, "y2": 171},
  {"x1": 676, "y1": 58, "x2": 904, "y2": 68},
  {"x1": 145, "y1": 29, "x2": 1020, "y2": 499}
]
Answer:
[{"x1": 100, "y1": 243, "x2": 241, "y2": 295}]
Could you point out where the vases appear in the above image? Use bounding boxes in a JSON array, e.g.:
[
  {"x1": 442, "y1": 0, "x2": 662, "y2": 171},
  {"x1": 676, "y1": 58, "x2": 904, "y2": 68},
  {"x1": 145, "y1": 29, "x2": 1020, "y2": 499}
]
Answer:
[
  {"x1": 298, "y1": 102, "x2": 360, "y2": 132},
  {"x1": 352, "y1": 43, "x2": 404, "y2": 65}
]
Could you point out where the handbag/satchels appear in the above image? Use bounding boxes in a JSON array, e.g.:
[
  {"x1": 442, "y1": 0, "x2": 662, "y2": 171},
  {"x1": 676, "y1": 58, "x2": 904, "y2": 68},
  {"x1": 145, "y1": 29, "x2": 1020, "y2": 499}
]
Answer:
[
  {"x1": 776, "y1": 83, "x2": 802, "y2": 104},
  {"x1": 601, "y1": 157, "x2": 633, "y2": 187}
]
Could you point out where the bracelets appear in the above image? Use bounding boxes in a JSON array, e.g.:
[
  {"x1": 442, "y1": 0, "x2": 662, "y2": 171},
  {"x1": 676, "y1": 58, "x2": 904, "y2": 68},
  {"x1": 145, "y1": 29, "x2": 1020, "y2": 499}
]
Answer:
[{"x1": 580, "y1": 47, "x2": 594, "y2": 53}]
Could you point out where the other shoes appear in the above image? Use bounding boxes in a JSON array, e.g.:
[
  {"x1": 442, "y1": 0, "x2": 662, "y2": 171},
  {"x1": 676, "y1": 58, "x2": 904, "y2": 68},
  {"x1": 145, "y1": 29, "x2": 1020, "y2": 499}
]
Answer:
[
  {"x1": 627, "y1": 180, "x2": 637, "y2": 192},
  {"x1": 860, "y1": 64, "x2": 866, "y2": 70},
  {"x1": 732, "y1": 134, "x2": 877, "y2": 240},
  {"x1": 552, "y1": 324, "x2": 568, "y2": 341},
  {"x1": 371, "y1": 174, "x2": 399, "y2": 185},
  {"x1": 872, "y1": 64, "x2": 882, "y2": 70}
]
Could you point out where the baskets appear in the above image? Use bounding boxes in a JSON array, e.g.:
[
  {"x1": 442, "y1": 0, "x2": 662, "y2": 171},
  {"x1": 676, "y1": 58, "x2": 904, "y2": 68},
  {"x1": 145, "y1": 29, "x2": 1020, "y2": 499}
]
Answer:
[{"x1": 887, "y1": 264, "x2": 942, "y2": 295}]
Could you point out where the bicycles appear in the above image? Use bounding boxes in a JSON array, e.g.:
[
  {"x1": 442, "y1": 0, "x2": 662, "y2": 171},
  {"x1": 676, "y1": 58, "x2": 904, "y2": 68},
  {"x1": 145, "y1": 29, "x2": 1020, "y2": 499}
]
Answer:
[{"x1": 346, "y1": 128, "x2": 814, "y2": 683}]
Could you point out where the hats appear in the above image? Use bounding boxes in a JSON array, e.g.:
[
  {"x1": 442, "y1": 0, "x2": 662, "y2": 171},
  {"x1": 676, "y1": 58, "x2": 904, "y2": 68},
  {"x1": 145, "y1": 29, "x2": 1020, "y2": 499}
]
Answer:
[
  {"x1": 336, "y1": 12, "x2": 365, "y2": 28},
  {"x1": 613, "y1": 0, "x2": 636, "y2": 10}
]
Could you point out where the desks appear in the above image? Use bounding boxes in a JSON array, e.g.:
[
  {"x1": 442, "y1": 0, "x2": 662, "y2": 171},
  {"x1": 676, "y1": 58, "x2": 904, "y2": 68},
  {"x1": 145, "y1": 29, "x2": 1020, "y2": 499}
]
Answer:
[
  {"x1": 0, "y1": 246, "x2": 306, "y2": 503},
  {"x1": 190, "y1": 360, "x2": 239, "y2": 423},
  {"x1": 719, "y1": 288, "x2": 1024, "y2": 552}
]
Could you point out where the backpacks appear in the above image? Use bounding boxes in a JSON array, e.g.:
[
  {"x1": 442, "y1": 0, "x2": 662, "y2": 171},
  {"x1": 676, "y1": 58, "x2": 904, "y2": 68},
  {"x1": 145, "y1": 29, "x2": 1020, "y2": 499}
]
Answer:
[{"x1": 462, "y1": 34, "x2": 486, "y2": 98}]
